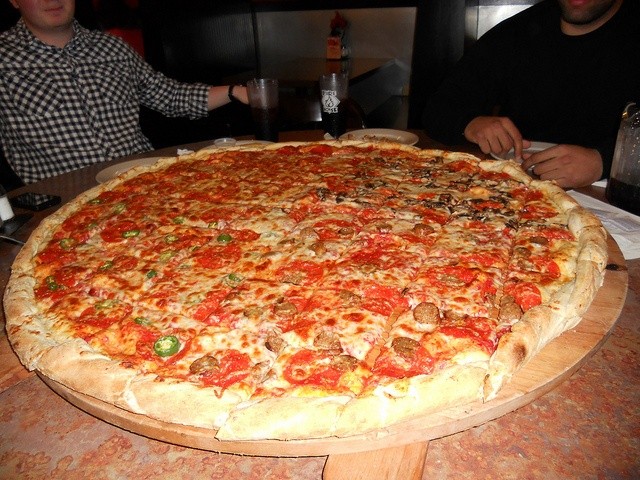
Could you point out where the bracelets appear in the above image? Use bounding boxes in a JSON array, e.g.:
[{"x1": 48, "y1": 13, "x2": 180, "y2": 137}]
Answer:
[{"x1": 228, "y1": 84, "x2": 237, "y2": 101}]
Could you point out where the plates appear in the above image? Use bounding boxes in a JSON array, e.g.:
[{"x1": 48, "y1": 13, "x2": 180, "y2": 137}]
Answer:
[
  {"x1": 204, "y1": 140, "x2": 273, "y2": 148},
  {"x1": 489, "y1": 141, "x2": 558, "y2": 171},
  {"x1": 95, "y1": 157, "x2": 169, "y2": 183},
  {"x1": 338, "y1": 127, "x2": 419, "y2": 146}
]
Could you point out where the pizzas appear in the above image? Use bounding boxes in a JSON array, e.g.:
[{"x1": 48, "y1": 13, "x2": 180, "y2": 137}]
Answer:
[{"x1": 3, "y1": 139, "x2": 608, "y2": 439}]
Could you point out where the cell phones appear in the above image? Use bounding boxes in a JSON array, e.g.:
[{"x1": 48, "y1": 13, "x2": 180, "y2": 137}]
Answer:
[{"x1": 10, "y1": 191, "x2": 61, "y2": 211}]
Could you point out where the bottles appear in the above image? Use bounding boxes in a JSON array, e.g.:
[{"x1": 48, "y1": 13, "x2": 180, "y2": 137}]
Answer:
[{"x1": 338, "y1": 45, "x2": 352, "y2": 80}]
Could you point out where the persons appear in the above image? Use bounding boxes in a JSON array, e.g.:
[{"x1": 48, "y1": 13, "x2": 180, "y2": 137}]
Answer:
[
  {"x1": 0, "y1": 0, "x2": 258, "y2": 185},
  {"x1": 422, "y1": 0, "x2": 640, "y2": 189}
]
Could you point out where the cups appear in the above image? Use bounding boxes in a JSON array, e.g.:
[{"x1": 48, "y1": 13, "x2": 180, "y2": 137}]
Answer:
[
  {"x1": 603, "y1": 103, "x2": 640, "y2": 217},
  {"x1": 214, "y1": 138, "x2": 236, "y2": 148},
  {"x1": 319, "y1": 73, "x2": 349, "y2": 140},
  {"x1": 247, "y1": 78, "x2": 279, "y2": 142}
]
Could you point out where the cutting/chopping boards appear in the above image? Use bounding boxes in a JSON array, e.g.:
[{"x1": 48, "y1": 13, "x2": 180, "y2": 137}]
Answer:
[{"x1": 34, "y1": 226, "x2": 629, "y2": 480}]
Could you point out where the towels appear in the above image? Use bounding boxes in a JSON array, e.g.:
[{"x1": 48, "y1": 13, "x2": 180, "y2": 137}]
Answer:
[{"x1": 565, "y1": 187, "x2": 639, "y2": 262}]
